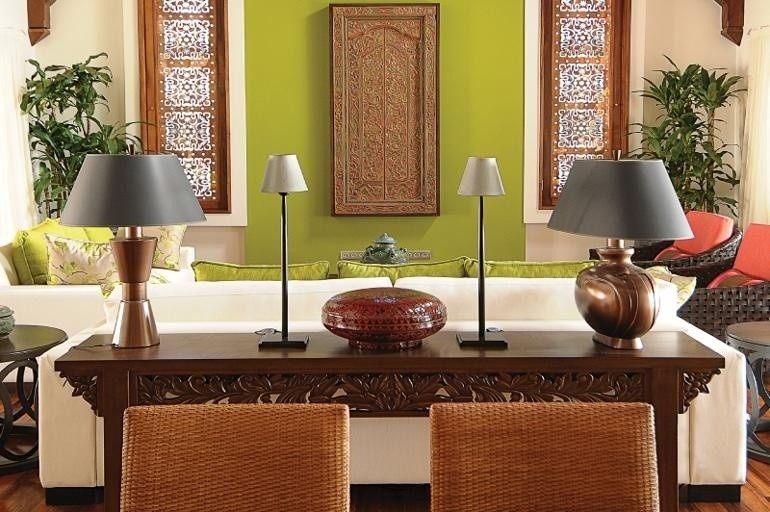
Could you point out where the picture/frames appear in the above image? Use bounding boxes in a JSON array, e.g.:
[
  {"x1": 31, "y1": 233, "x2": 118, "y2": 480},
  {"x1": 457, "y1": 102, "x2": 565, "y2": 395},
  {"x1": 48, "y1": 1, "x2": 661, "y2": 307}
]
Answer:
[{"x1": 329, "y1": 3, "x2": 440, "y2": 218}]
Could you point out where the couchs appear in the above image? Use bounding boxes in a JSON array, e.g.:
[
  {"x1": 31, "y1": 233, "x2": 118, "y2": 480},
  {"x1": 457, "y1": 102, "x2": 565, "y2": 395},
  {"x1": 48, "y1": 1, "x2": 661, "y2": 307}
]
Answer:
[{"x1": 36, "y1": 274, "x2": 747, "y2": 504}]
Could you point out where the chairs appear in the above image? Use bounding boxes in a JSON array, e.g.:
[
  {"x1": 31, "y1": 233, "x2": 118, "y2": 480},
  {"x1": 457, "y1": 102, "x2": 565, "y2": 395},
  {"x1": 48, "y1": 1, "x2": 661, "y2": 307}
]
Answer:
[
  {"x1": 630, "y1": 226, "x2": 743, "y2": 288},
  {"x1": 119, "y1": 401, "x2": 350, "y2": 512},
  {"x1": 668, "y1": 254, "x2": 770, "y2": 344},
  {"x1": 428, "y1": 401, "x2": 660, "y2": 512},
  {"x1": 0, "y1": 240, "x2": 195, "y2": 384}
]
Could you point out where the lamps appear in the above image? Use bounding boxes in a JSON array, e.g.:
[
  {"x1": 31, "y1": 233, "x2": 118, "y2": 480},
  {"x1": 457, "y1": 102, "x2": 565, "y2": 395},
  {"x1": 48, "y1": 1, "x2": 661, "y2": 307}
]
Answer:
[
  {"x1": 58, "y1": 144, "x2": 207, "y2": 349},
  {"x1": 257, "y1": 152, "x2": 310, "y2": 351},
  {"x1": 545, "y1": 149, "x2": 696, "y2": 350},
  {"x1": 455, "y1": 156, "x2": 509, "y2": 350}
]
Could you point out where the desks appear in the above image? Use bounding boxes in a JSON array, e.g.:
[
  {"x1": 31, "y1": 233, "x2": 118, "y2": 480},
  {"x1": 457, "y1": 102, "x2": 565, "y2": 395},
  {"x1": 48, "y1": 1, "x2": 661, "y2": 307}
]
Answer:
[
  {"x1": 53, "y1": 330, "x2": 725, "y2": 512},
  {"x1": 724, "y1": 320, "x2": 770, "y2": 464},
  {"x1": 0, "y1": 324, "x2": 68, "y2": 476},
  {"x1": 588, "y1": 248, "x2": 599, "y2": 259}
]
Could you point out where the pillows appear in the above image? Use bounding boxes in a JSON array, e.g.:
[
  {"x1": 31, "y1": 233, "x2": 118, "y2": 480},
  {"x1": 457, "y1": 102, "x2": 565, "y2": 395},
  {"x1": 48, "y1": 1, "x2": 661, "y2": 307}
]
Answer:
[
  {"x1": 652, "y1": 245, "x2": 694, "y2": 261},
  {"x1": 644, "y1": 265, "x2": 697, "y2": 312},
  {"x1": 54, "y1": 217, "x2": 117, "y2": 242},
  {"x1": 116, "y1": 224, "x2": 189, "y2": 271},
  {"x1": 706, "y1": 268, "x2": 765, "y2": 289},
  {"x1": 42, "y1": 233, "x2": 123, "y2": 300},
  {"x1": 10, "y1": 217, "x2": 68, "y2": 285}
]
